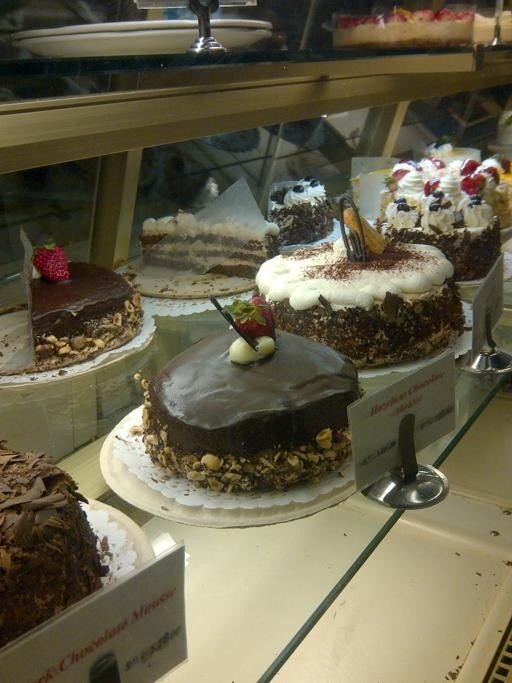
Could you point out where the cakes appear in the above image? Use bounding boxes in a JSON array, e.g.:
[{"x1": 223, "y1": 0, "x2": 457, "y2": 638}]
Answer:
[
  {"x1": 0, "y1": 437, "x2": 113, "y2": 647},
  {"x1": 267, "y1": 110, "x2": 512, "y2": 281},
  {"x1": 138, "y1": 211, "x2": 283, "y2": 280},
  {"x1": 130, "y1": 294, "x2": 368, "y2": 493},
  {"x1": 252, "y1": 194, "x2": 467, "y2": 369},
  {"x1": 25, "y1": 239, "x2": 144, "y2": 371},
  {"x1": 331, "y1": 8, "x2": 475, "y2": 51}
]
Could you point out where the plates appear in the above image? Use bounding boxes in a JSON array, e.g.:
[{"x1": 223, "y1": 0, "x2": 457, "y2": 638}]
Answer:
[{"x1": 7, "y1": 16, "x2": 276, "y2": 57}]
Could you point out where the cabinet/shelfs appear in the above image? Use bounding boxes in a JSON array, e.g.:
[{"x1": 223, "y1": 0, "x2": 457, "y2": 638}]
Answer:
[{"x1": 0, "y1": 40, "x2": 512, "y2": 683}]
